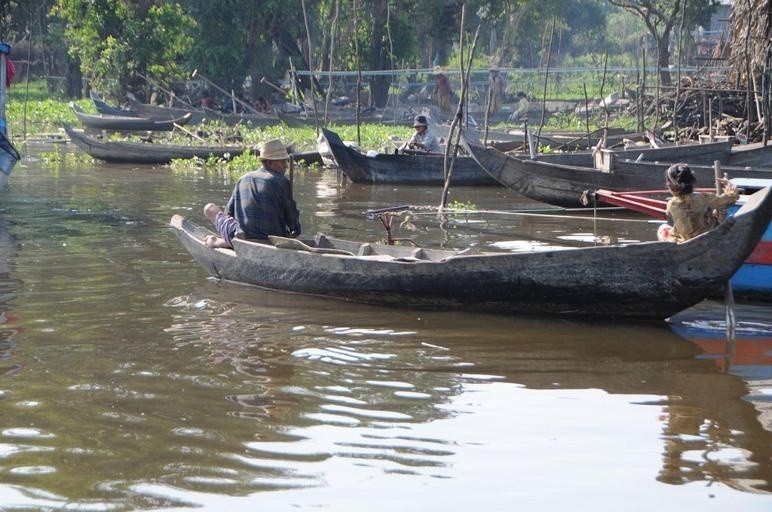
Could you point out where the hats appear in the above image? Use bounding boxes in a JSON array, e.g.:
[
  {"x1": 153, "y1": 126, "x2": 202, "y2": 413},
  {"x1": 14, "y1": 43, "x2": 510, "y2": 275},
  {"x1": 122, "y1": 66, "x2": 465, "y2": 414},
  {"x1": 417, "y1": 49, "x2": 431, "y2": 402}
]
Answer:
[
  {"x1": 257, "y1": 139, "x2": 290, "y2": 162},
  {"x1": 412, "y1": 116, "x2": 427, "y2": 128}
]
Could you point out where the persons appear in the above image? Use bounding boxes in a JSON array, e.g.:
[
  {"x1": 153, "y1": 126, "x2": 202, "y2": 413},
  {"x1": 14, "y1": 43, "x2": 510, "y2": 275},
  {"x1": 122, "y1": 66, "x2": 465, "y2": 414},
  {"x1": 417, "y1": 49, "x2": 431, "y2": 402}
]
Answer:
[
  {"x1": 201, "y1": 138, "x2": 301, "y2": 250},
  {"x1": 656, "y1": 160, "x2": 738, "y2": 244},
  {"x1": 408, "y1": 114, "x2": 443, "y2": 154},
  {"x1": 433, "y1": 71, "x2": 454, "y2": 113},
  {"x1": 198, "y1": 89, "x2": 288, "y2": 114},
  {"x1": 511, "y1": 92, "x2": 530, "y2": 121}
]
